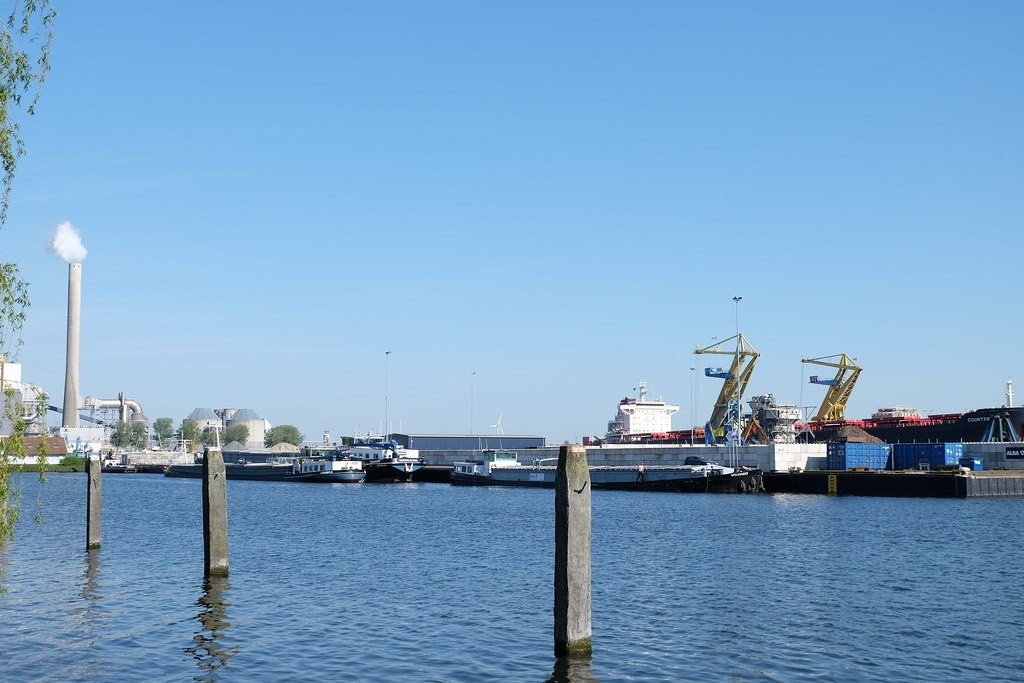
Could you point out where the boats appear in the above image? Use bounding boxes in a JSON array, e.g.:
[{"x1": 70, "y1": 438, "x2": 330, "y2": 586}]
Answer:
[
  {"x1": 164, "y1": 463, "x2": 294, "y2": 481},
  {"x1": 283, "y1": 455, "x2": 366, "y2": 482},
  {"x1": 448, "y1": 449, "x2": 763, "y2": 492},
  {"x1": 102, "y1": 460, "x2": 139, "y2": 473}
]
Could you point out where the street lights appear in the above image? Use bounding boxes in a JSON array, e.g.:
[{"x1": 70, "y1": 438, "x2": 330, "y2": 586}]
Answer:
[
  {"x1": 386, "y1": 351, "x2": 391, "y2": 442},
  {"x1": 733, "y1": 296, "x2": 743, "y2": 446}
]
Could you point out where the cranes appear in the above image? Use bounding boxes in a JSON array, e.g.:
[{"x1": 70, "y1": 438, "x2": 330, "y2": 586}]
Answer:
[
  {"x1": 692, "y1": 332, "x2": 761, "y2": 430},
  {"x1": 800, "y1": 353, "x2": 863, "y2": 420}
]
